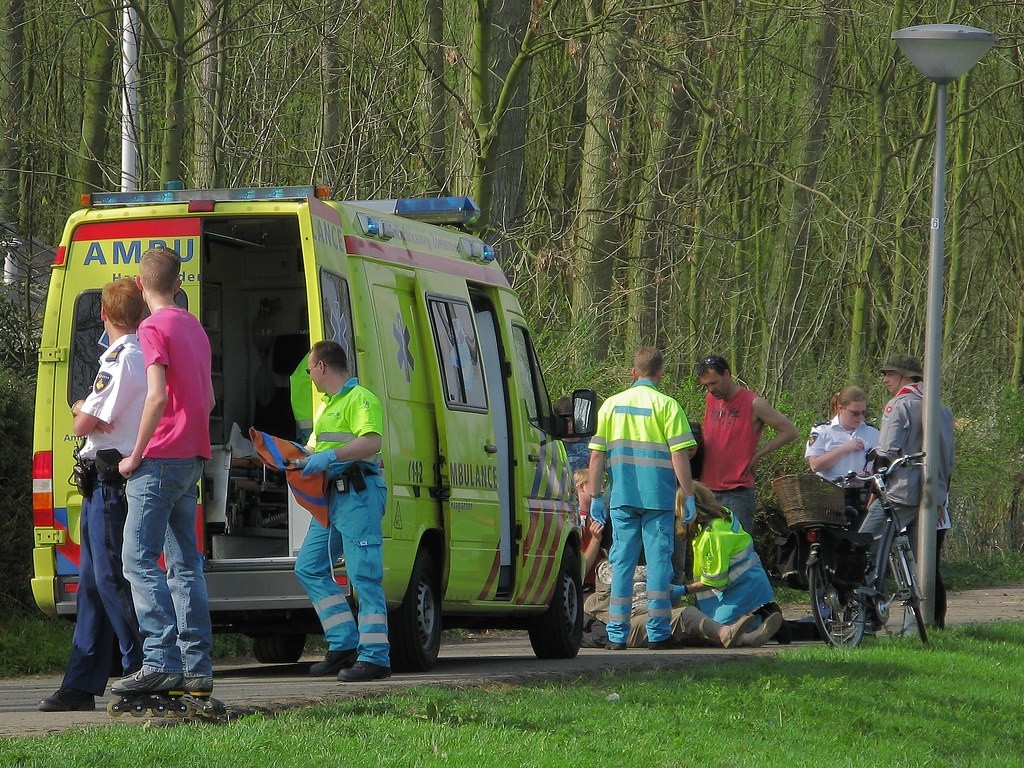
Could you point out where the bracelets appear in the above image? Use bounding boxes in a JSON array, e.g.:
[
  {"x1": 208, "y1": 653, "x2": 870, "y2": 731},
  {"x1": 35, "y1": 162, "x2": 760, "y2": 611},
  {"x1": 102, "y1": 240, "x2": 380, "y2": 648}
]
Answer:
[
  {"x1": 70, "y1": 400, "x2": 80, "y2": 413},
  {"x1": 590, "y1": 494, "x2": 600, "y2": 499}
]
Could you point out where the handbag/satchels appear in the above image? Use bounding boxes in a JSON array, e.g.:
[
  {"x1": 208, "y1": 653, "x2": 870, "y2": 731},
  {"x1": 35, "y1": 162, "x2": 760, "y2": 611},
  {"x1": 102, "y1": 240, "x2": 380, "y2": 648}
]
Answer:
[{"x1": 776, "y1": 524, "x2": 874, "y2": 591}]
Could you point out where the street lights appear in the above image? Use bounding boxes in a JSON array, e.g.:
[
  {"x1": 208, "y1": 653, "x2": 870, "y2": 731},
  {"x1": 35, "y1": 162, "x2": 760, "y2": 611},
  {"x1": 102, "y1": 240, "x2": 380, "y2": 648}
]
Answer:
[{"x1": 888, "y1": 19, "x2": 994, "y2": 628}]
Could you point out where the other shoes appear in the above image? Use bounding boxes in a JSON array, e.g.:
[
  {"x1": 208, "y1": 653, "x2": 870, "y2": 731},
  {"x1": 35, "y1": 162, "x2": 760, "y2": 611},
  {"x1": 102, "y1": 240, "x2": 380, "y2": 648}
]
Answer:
[
  {"x1": 721, "y1": 614, "x2": 755, "y2": 648},
  {"x1": 744, "y1": 612, "x2": 782, "y2": 648},
  {"x1": 649, "y1": 636, "x2": 683, "y2": 650},
  {"x1": 604, "y1": 640, "x2": 626, "y2": 649}
]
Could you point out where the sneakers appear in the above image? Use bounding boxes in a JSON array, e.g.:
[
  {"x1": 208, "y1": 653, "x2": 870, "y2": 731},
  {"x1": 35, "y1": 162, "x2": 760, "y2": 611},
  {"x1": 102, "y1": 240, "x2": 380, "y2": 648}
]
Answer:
[
  {"x1": 38, "y1": 684, "x2": 94, "y2": 712},
  {"x1": 308, "y1": 648, "x2": 359, "y2": 677},
  {"x1": 337, "y1": 660, "x2": 391, "y2": 681}
]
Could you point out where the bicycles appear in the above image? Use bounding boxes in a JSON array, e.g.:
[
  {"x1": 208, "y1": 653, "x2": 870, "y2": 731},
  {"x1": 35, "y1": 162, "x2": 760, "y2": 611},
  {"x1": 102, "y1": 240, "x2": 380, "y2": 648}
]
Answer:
[{"x1": 805, "y1": 446, "x2": 929, "y2": 651}]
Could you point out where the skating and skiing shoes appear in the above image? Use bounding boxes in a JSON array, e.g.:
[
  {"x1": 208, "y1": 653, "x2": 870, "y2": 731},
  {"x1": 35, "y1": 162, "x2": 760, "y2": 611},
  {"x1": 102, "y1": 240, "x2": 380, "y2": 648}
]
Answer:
[
  {"x1": 107, "y1": 667, "x2": 183, "y2": 717},
  {"x1": 166, "y1": 674, "x2": 212, "y2": 721}
]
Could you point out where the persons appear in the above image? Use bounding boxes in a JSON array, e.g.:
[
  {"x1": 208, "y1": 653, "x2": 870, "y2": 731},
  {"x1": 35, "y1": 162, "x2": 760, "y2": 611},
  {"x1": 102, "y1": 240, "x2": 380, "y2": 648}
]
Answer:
[
  {"x1": 859, "y1": 354, "x2": 955, "y2": 628},
  {"x1": 697, "y1": 355, "x2": 798, "y2": 534},
  {"x1": 672, "y1": 479, "x2": 791, "y2": 645},
  {"x1": 574, "y1": 468, "x2": 608, "y2": 649},
  {"x1": 689, "y1": 422, "x2": 705, "y2": 478},
  {"x1": 804, "y1": 386, "x2": 881, "y2": 621},
  {"x1": 588, "y1": 346, "x2": 698, "y2": 650},
  {"x1": 37, "y1": 278, "x2": 148, "y2": 710},
  {"x1": 584, "y1": 515, "x2": 783, "y2": 649},
  {"x1": 556, "y1": 394, "x2": 592, "y2": 471},
  {"x1": 107, "y1": 247, "x2": 215, "y2": 718},
  {"x1": 292, "y1": 341, "x2": 391, "y2": 682}
]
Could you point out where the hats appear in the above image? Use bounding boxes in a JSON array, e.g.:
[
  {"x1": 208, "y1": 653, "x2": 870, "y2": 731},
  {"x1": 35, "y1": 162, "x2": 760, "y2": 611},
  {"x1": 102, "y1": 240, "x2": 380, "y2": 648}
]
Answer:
[{"x1": 880, "y1": 353, "x2": 923, "y2": 378}]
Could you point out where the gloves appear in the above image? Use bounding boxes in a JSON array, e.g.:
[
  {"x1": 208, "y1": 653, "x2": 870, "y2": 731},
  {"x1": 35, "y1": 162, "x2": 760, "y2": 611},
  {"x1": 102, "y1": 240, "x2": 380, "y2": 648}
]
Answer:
[
  {"x1": 301, "y1": 450, "x2": 337, "y2": 476},
  {"x1": 680, "y1": 495, "x2": 695, "y2": 525},
  {"x1": 590, "y1": 495, "x2": 607, "y2": 525}
]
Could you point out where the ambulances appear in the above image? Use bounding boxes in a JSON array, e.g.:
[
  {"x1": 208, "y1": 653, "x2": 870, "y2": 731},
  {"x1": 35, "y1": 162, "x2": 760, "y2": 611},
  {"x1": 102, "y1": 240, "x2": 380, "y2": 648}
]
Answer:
[{"x1": 33, "y1": 185, "x2": 599, "y2": 673}]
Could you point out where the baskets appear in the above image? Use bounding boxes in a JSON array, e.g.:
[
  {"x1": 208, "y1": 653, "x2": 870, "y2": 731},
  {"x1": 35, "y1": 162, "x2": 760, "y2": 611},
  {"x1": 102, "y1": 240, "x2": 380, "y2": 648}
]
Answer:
[{"x1": 771, "y1": 473, "x2": 846, "y2": 528}]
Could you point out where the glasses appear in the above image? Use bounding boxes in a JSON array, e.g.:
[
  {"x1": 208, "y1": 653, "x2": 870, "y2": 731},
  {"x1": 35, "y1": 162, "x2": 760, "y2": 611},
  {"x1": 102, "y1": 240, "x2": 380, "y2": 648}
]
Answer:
[
  {"x1": 305, "y1": 360, "x2": 328, "y2": 374},
  {"x1": 698, "y1": 358, "x2": 726, "y2": 369},
  {"x1": 840, "y1": 404, "x2": 866, "y2": 416}
]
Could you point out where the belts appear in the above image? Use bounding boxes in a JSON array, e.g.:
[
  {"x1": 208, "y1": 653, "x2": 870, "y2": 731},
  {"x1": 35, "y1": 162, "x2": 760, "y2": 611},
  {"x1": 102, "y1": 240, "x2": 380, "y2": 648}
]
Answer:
[{"x1": 327, "y1": 470, "x2": 377, "y2": 489}]
